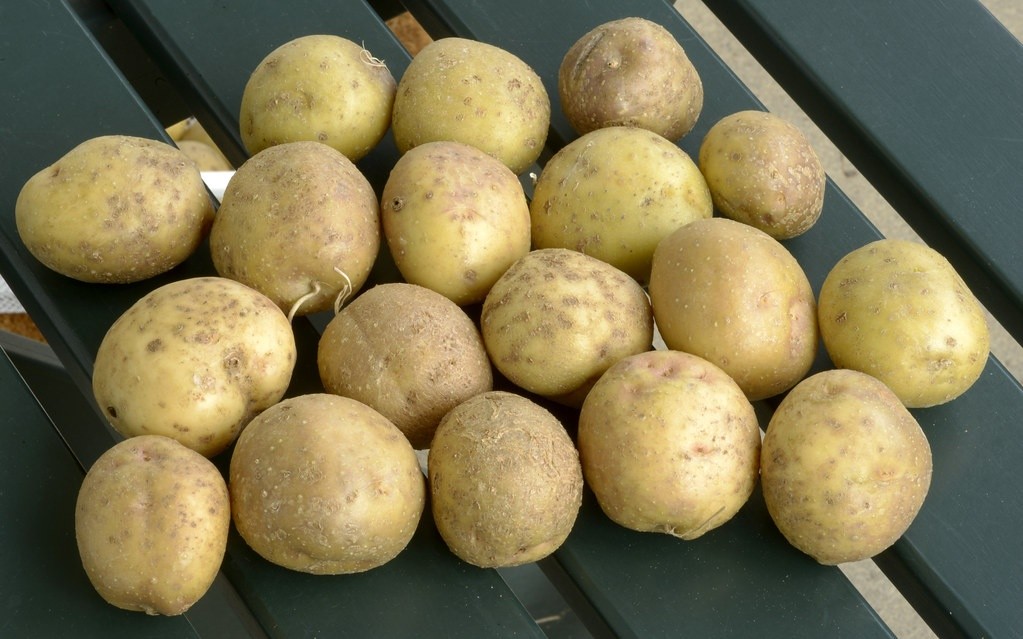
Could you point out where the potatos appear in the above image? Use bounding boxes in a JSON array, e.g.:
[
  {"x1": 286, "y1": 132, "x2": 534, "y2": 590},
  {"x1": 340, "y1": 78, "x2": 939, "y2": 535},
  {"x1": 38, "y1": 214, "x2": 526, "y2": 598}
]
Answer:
[{"x1": 16, "y1": 18, "x2": 990, "y2": 614}]
